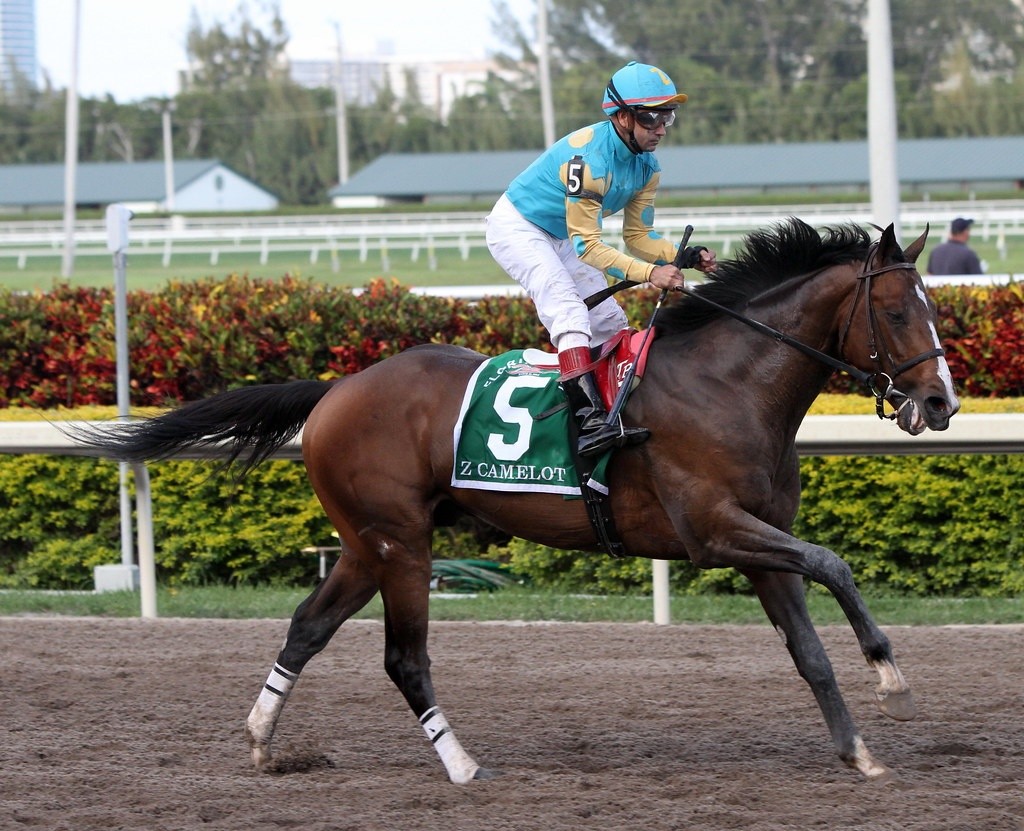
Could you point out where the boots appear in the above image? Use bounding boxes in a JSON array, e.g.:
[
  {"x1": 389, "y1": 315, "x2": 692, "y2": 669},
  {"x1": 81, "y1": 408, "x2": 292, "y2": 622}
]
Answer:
[{"x1": 559, "y1": 346, "x2": 648, "y2": 456}]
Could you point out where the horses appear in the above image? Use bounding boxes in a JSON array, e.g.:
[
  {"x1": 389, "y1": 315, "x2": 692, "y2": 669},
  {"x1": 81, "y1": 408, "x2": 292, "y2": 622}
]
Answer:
[{"x1": 31, "y1": 212, "x2": 962, "y2": 787}]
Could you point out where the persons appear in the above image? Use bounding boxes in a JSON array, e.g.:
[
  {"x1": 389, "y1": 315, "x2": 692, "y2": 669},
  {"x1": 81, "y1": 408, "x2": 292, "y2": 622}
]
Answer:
[
  {"x1": 485, "y1": 61, "x2": 722, "y2": 456},
  {"x1": 927, "y1": 218, "x2": 983, "y2": 274}
]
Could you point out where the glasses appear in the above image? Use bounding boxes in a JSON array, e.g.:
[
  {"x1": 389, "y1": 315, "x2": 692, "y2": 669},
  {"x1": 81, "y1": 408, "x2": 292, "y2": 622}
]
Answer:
[{"x1": 636, "y1": 111, "x2": 676, "y2": 129}]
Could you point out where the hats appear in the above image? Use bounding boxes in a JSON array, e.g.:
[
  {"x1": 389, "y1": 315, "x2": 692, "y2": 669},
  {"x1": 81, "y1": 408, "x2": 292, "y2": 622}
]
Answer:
[
  {"x1": 952, "y1": 218, "x2": 973, "y2": 232},
  {"x1": 602, "y1": 61, "x2": 688, "y2": 112}
]
